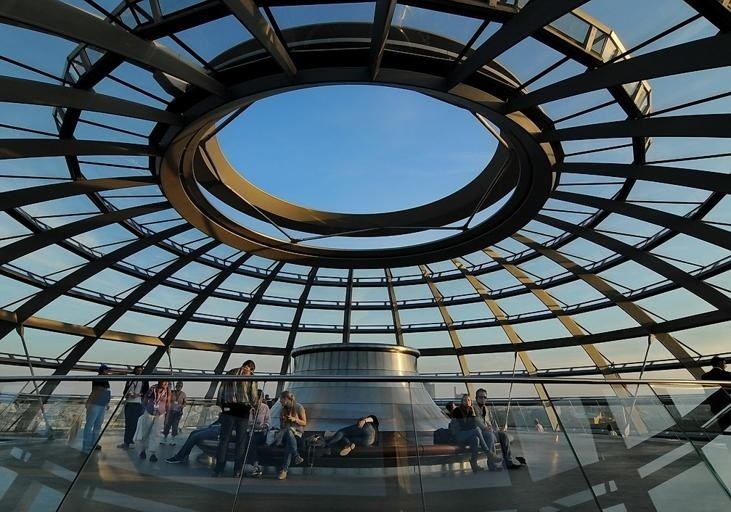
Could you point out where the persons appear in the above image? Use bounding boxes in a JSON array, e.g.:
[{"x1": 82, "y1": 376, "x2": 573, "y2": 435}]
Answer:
[
  {"x1": 117, "y1": 365, "x2": 149, "y2": 448},
  {"x1": 274, "y1": 391, "x2": 307, "y2": 480},
  {"x1": 444, "y1": 388, "x2": 522, "y2": 473},
  {"x1": 534, "y1": 417, "x2": 544, "y2": 432},
  {"x1": 166, "y1": 360, "x2": 270, "y2": 478},
  {"x1": 160, "y1": 381, "x2": 186, "y2": 446},
  {"x1": 319, "y1": 416, "x2": 379, "y2": 457},
  {"x1": 82, "y1": 365, "x2": 110, "y2": 454},
  {"x1": 140, "y1": 380, "x2": 171, "y2": 462}
]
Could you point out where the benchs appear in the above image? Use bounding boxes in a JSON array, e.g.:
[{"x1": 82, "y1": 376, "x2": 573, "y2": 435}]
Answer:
[
  {"x1": 196, "y1": 431, "x2": 515, "y2": 476},
  {"x1": 554, "y1": 417, "x2": 617, "y2": 436}
]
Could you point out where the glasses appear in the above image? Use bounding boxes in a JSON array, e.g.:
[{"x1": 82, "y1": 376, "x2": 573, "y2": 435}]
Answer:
[{"x1": 479, "y1": 395, "x2": 488, "y2": 399}]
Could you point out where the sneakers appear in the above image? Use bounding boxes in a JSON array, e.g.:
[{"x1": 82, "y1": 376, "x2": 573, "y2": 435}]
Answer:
[
  {"x1": 138, "y1": 450, "x2": 158, "y2": 462},
  {"x1": 487, "y1": 451, "x2": 502, "y2": 471},
  {"x1": 164, "y1": 456, "x2": 188, "y2": 463},
  {"x1": 340, "y1": 442, "x2": 355, "y2": 457},
  {"x1": 117, "y1": 442, "x2": 137, "y2": 449},
  {"x1": 251, "y1": 465, "x2": 262, "y2": 479},
  {"x1": 214, "y1": 469, "x2": 241, "y2": 478},
  {"x1": 277, "y1": 469, "x2": 286, "y2": 480},
  {"x1": 159, "y1": 437, "x2": 177, "y2": 446},
  {"x1": 470, "y1": 462, "x2": 483, "y2": 472}
]
